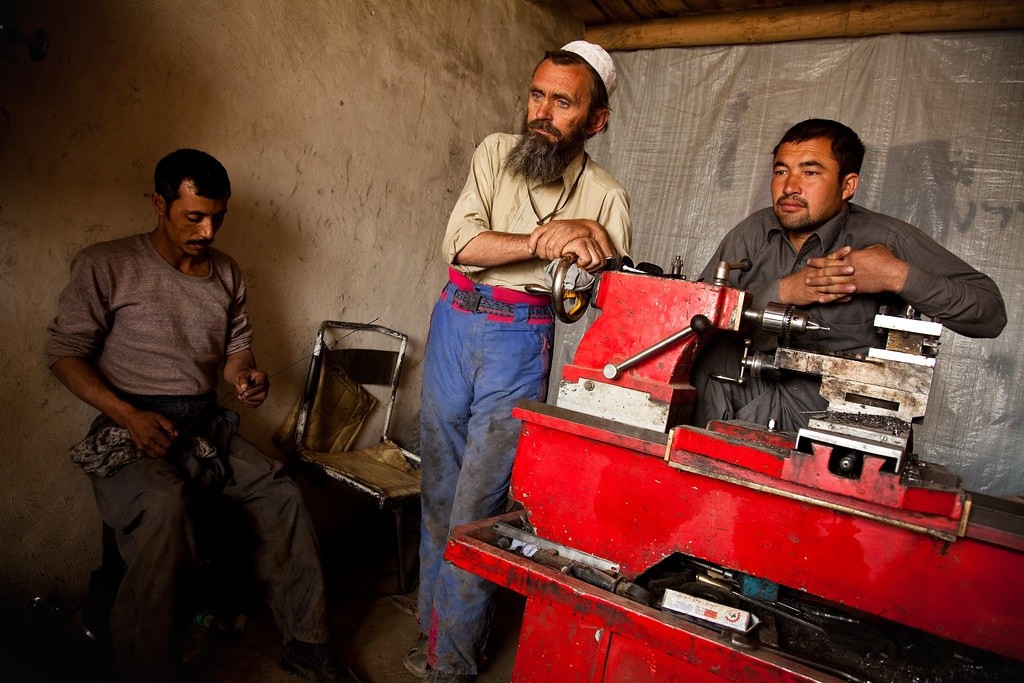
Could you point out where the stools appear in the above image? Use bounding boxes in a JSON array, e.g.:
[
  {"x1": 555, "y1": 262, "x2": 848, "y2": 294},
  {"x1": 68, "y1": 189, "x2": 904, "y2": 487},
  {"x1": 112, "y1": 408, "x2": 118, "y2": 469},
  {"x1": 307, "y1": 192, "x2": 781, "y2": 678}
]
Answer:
[{"x1": 92, "y1": 485, "x2": 235, "y2": 627}]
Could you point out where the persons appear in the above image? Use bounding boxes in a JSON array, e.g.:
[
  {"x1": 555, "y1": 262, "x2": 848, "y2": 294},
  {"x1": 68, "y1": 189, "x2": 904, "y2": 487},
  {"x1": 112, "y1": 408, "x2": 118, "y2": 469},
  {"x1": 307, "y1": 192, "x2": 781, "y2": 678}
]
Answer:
[
  {"x1": 45, "y1": 148, "x2": 359, "y2": 683},
  {"x1": 403, "y1": 41, "x2": 633, "y2": 683},
  {"x1": 689, "y1": 119, "x2": 1007, "y2": 429}
]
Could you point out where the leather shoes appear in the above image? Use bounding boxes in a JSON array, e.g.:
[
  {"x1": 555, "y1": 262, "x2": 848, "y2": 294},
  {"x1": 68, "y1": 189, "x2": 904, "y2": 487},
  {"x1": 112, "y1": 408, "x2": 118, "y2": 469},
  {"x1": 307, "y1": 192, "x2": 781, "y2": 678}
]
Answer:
[{"x1": 279, "y1": 639, "x2": 362, "y2": 683}]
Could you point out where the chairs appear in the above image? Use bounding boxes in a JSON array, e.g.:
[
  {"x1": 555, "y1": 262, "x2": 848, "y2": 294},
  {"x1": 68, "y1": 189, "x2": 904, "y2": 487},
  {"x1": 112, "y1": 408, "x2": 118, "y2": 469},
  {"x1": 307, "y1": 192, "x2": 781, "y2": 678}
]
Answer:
[{"x1": 281, "y1": 320, "x2": 425, "y2": 598}]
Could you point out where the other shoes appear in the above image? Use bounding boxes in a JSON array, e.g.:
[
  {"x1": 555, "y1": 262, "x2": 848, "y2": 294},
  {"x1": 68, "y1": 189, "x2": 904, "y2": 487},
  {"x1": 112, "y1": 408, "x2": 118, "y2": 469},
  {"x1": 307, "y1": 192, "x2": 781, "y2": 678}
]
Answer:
[
  {"x1": 402, "y1": 632, "x2": 434, "y2": 678},
  {"x1": 421, "y1": 659, "x2": 479, "y2": 683}
]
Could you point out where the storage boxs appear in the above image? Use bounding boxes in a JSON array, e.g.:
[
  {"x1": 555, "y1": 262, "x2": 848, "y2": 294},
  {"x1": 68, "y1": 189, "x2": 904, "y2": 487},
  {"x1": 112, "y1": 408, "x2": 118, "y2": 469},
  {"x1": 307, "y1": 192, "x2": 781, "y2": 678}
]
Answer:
[{"x1": 661, "y1": 589, "x2": 762, "y2": 633}]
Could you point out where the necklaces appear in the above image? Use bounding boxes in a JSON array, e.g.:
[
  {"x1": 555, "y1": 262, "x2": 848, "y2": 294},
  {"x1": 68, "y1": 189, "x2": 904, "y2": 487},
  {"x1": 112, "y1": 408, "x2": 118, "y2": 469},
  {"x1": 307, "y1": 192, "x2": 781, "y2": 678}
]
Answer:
[{"x1": 525, "y1": 150, "x2": 588, "y2": 225}]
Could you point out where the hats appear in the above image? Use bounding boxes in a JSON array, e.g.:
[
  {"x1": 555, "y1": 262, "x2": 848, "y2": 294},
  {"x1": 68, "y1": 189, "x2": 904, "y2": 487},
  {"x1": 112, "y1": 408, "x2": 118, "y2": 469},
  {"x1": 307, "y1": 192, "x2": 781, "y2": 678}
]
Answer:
[{"x1": 560, "y1": 40, "x2": 618, "y2": 99}]
